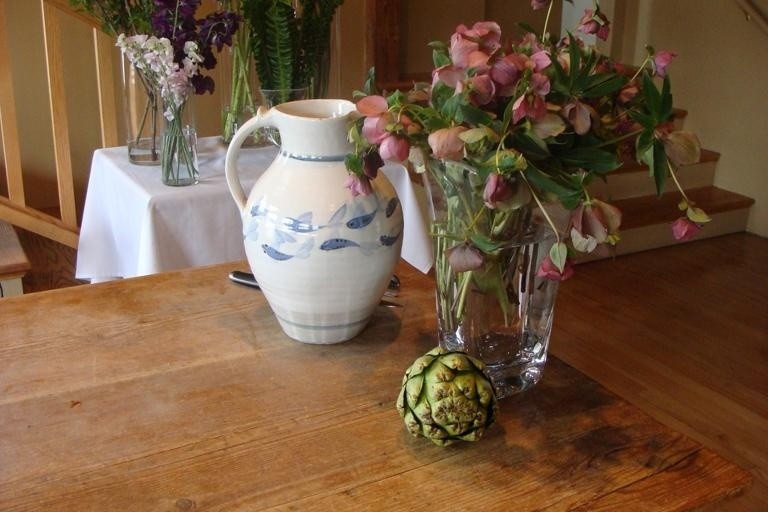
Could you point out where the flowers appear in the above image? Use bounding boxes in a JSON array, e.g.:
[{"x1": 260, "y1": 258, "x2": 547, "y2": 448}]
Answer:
[
  {"x1": 115, "y1": 1, "x2": 242, "y2": 183},
  {"x1": 343, "y1": 1, "x2": 713, "y2": 334}
]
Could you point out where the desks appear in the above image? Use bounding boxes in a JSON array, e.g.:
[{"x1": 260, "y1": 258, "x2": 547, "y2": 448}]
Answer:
[
  {"x1": 95, "y1": 135, "x2": 403, "y2": 274},
  {"x1": 0, "y1": 253, "x2": 768, "y2": 512}
]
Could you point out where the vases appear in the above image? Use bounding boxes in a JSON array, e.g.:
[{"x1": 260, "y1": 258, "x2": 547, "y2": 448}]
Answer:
[
  {"x1": 218, "y1": 0, "x2": 273, "y2": 148},
  {"x1": 421, "y1": 154, "x2": 593, "y2": 400},
  {"x1": 156, "y1": 84, "x2": 201, "y2": 187}
]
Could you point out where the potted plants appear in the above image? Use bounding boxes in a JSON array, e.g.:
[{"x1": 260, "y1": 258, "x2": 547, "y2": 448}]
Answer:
[
  {"x1": 240, "y1": 0, "x2": 345, "y2": 148},
  {"x1": 65, "y1": 1, "x2": 164, "y2": 165}
]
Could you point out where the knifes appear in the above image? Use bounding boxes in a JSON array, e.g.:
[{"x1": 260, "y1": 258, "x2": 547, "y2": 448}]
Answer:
[{"x1": 226, "y1": 266, "x2": 404, "y2": 310}]
